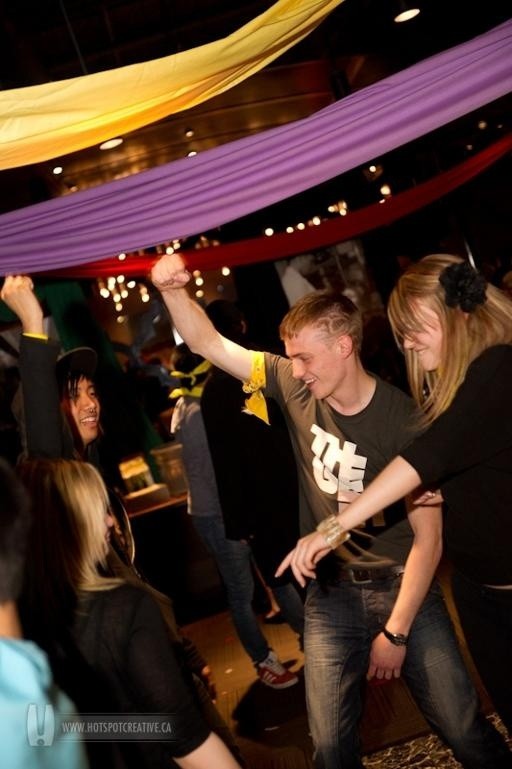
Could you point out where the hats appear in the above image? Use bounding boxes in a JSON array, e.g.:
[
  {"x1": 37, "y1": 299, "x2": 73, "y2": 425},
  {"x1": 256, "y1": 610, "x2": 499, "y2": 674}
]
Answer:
[{"x1": 11, "y1": 346, "x2": 96, "y2": 419}]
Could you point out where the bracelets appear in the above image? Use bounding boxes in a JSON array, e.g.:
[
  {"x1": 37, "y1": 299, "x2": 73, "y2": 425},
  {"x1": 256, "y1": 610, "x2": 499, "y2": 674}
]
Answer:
[
  {"x1": 23, "y1": 332, "x2": 49, "y2": 340},
  {"x1": 316, "y1": 513, "x2": 352, "y2": 548}
]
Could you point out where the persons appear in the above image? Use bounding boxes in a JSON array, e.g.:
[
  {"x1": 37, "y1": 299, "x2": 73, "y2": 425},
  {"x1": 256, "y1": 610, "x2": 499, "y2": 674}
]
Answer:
[
  {"x1": 251, "y1": 558, "x2": 286, "y2": 625},
  {"x1": 200, "y1": 298, "x2": 304, "y2": 636},
  {"x1": 1, "y1": 459, "x2": 89, "y2": 769},
  {"x1": 341, "y1": 283, "x2": 408, "y2": 392},
  {"x1": 275, "y1": 253, "x2": 511, "y2": 741},
  {"x1": 0, "y1": 276, "x2": 142, "y2": 574},
  {"x1": 168, "y1": 344, "x2": 304, "y2": 688},
  {"x1": 343, "y1": 261, "x2": 381, "y2": 308},
  {"x1": 148, "y1": 253, "x2": 512, "y2": 769},
  {"x1": 32, "y1": 461, "x2": 240, "y2": 769}
]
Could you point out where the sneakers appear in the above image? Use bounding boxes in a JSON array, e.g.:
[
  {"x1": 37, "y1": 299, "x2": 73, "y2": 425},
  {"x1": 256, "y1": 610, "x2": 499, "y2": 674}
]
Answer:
[{"x1": 254, "y1": 649, "x2": 297, "y2": 689}]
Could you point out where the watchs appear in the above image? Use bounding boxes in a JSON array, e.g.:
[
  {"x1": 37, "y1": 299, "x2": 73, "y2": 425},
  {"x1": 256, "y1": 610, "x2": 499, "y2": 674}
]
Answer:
[{"x1": 382, "y1": 628, "x2": 410, "y2": 651}]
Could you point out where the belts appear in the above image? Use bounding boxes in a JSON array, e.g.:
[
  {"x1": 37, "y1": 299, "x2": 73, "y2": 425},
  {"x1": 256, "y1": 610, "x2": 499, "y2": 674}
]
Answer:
[{"x1": 333, "y1": 563, "x2": 403, "y2": 585}]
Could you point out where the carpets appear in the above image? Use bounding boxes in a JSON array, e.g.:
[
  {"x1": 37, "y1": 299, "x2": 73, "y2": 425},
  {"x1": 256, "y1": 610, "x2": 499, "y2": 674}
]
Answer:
[{"x1": 360, "y1": 710, "x2": 512, "y2": 769}]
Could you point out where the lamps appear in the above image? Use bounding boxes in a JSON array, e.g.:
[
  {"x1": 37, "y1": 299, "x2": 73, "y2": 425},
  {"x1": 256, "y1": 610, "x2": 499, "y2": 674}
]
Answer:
[
  {"x1": 60, "y1": 0, "x2": 124, "y2": 151},
  {"x1": 393, "y1": 0, "x2": 421, "y2": 23}
]
